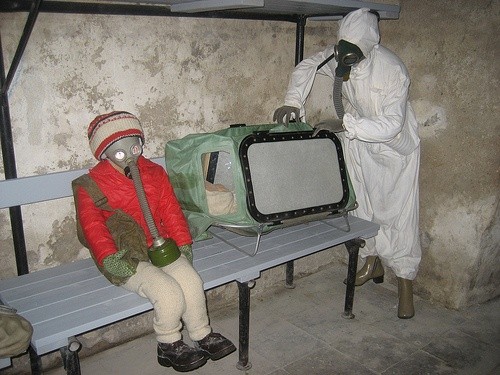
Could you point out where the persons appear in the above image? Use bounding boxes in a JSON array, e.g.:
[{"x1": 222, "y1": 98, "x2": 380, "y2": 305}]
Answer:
[
  {"x1": 70, "y1": 110, "x2": 237, "y2": 372},
  {"x1": 272, "y1": 7, "x2": 422, "y2": 319}
]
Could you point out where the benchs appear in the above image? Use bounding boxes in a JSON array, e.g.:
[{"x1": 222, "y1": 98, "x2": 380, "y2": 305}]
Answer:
[{"x1": 0, "y1": 155, "x2": 379, "y2": 375}]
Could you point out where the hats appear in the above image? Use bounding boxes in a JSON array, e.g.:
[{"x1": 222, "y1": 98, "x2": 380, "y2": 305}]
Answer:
[{"x1": 88, "y1": 110, "x2": 146, "y2": 161}]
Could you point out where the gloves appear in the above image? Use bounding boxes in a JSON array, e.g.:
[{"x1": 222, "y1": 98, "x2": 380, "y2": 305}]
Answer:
[
  {"x1": 273, "y1": 105, "x2": 301, "y2": 128},
  {"x1": 104, "y1": 249, "x2": 135, "y2": 278},
  {"x1": 313, "y1": 118, "x2": 345, "y2": 138},
  {"x1": 180, "y1": 243, "x2": 194, "y2": 265}
]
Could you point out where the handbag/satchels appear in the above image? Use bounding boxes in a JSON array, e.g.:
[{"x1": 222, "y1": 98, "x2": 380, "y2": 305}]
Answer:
[
  {"x1": 71, "y1": 173, "x2": 150, "y2": 287},
  {"x1": 0, "y1": 294, "x2": 34, "y2": 358}
]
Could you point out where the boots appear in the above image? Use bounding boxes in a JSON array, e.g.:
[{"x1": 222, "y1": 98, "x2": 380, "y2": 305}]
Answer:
[
  {"x1": 398, "y1": 278, "x2": 414, "y2": 319},
  {"x1": 343, "y1": 255, "x2": 384, "y2": 285}
]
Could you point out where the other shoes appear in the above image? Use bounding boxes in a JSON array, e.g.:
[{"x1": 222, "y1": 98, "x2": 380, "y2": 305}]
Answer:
[
  {"x1": 156, "y1": 340, "x2": 207, "y2": 372},
  {"x1": 194, "y1": 332, "x2": 237, "y2": 362}
]
